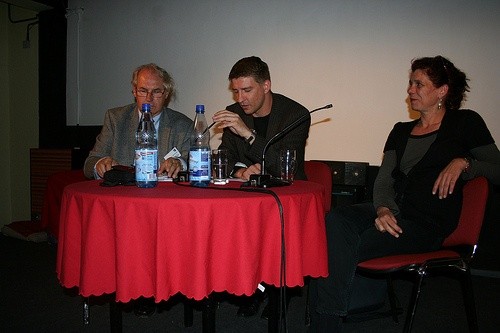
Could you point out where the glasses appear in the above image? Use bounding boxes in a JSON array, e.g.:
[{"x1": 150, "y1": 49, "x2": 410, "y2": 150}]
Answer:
[{"x1": 135, "y1": 89, "x2": 165, "y2": 98}]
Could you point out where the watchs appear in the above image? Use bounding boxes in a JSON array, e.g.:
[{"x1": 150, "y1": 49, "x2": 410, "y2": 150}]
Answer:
[{"x1": 245, "y1": 128, "x2": 257, "y2": 143}]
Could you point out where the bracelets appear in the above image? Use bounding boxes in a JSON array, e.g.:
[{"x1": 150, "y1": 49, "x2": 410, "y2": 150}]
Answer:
[{"x1": 462, "y1": 155, "x2": 472, "y2": 172}]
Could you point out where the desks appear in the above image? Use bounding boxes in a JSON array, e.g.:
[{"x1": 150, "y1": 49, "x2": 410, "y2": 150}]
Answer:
[{"x1": 57, "y1": 180, "x2": 329, "y2": 333}]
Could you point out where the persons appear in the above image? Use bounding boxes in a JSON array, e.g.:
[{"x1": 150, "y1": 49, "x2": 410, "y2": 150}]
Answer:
[
  {"x1": 309, "y1": 55, "x2": 500, "y2": 333},
  {"x1": 83, "y1": 62, "x2": 194, "y2": 320},
  {"x1": 211, "y1": 56, "x2": 311, "y2": 320}
]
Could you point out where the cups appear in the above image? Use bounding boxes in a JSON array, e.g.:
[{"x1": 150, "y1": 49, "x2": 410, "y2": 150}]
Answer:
[
  {"x1": 280, "y1": 149, "x2": 297, "y2": 184},
  {"x1": 212, "y1": 149, "x2": 228, "y2": 185}
]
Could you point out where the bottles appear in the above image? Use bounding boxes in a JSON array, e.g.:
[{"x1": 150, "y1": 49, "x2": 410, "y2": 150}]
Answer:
[
  {"x1": 187, "y1": 105, "x2": 212, "y2": 187},
  {"x1": 134, "y1": 103, "x2": 159, "y2": 188}
]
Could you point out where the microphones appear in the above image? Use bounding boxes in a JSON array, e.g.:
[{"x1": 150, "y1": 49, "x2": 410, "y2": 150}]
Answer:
[
  {"x1": 176, "y1": 121, "x2": 217, "y2": 181},
  {"x1": 250, "y1": 104, "x2": 332, "y2": 186}
]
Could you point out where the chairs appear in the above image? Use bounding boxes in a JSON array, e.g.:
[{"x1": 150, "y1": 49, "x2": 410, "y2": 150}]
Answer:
[
  {"x1": 47, "y1": 169, "x2": 88, "y2": 247},
  {"x1": 356, "y1": 176, "x2": 488, "y2": 333},
  {"x1": 302, "y1": 162, "x2": 332, "y2": 213}
]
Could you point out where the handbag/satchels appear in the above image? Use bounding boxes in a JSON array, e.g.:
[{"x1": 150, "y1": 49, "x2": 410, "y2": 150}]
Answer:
[{"x1": 99, "y1": 166, "x2": 136, "y2": 186}]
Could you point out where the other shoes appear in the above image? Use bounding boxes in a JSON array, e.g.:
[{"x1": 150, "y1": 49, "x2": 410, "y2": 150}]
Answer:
[
  {"x1": 262, "y1": 296, "x2": 290, "y2": 320},
  {"x1": 134, "y1": 303, "x2": 156, "y2": 319},
  {"x1": 237, "y1": 301, "x2": 260, "y2": 317}
]
